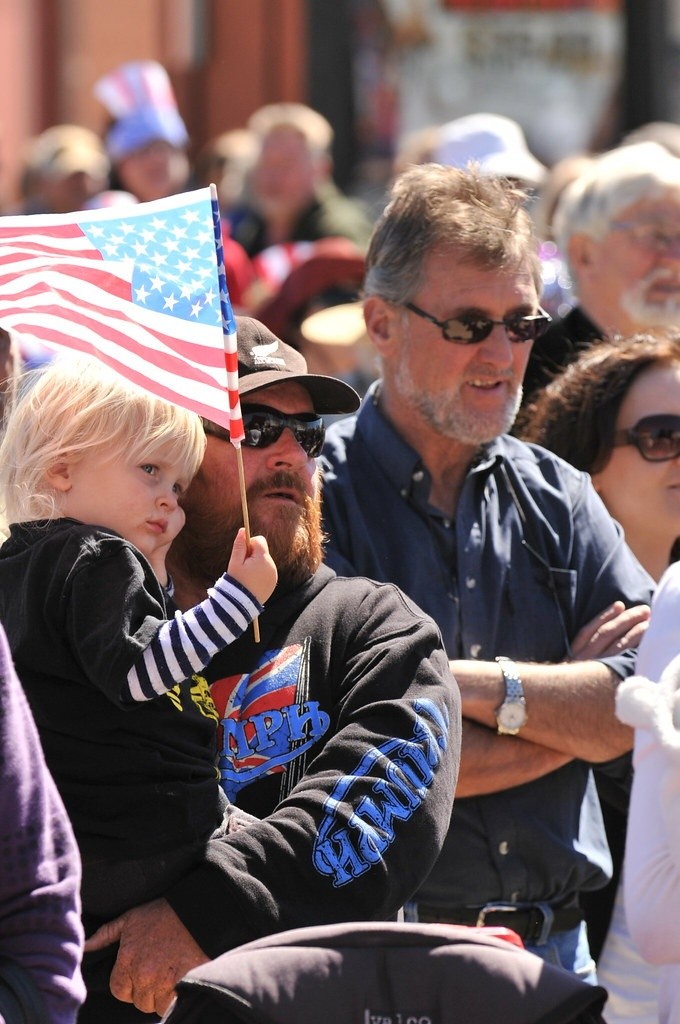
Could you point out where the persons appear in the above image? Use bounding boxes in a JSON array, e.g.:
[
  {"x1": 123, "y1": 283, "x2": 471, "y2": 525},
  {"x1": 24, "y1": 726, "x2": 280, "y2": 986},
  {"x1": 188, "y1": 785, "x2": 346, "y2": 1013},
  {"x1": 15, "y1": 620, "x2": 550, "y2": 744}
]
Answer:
[{"x1": 0, "y1": 61, "x2": 680, "y2": 1024}]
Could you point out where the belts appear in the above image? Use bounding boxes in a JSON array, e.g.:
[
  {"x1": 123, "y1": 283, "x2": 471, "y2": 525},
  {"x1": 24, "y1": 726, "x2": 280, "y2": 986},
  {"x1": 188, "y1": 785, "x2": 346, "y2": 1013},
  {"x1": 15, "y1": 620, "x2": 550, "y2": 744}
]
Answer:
[{"x1": 394, "y1": 892, "x2": 583, "y2": 945}]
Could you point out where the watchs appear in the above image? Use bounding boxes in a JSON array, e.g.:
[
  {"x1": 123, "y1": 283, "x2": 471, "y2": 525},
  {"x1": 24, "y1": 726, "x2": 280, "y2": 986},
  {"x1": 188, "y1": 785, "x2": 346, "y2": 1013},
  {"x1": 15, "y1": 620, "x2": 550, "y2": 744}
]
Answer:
[{"x1": 496, "y1": 657, "x2": 527, "y2": 736}]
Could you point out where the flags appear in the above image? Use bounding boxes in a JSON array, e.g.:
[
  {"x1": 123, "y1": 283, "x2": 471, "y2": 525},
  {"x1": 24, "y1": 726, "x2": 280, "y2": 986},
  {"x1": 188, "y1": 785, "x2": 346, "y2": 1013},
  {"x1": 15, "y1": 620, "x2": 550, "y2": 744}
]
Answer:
[{"x1": 0, "y1": 183, "x2": 247, "y2": 445}]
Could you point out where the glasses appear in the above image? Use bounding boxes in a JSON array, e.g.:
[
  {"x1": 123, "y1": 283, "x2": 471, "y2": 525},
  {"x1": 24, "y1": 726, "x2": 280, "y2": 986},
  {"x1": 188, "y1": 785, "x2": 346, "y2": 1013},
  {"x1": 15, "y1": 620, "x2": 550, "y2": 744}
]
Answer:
[
  {"x1": 403, "y1": 301, "x2": 553, "y2": 345},
  {"x1": 604, "y1": 415, "x2": 680, "y2": 463},
  {"x1": 201, "y1": 401, "x2": 327, "y2": 458}
]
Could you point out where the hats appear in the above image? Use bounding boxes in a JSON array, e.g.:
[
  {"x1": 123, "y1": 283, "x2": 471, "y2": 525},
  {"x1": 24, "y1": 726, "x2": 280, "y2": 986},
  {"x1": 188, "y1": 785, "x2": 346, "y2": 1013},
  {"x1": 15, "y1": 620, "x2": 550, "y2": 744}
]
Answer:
[
  {"x1": 235, "y1": 315, "x2": 362, "y2": 415},
  {"x1": 434, "y1": 113, "x2": 546, "y2": 180}
]
